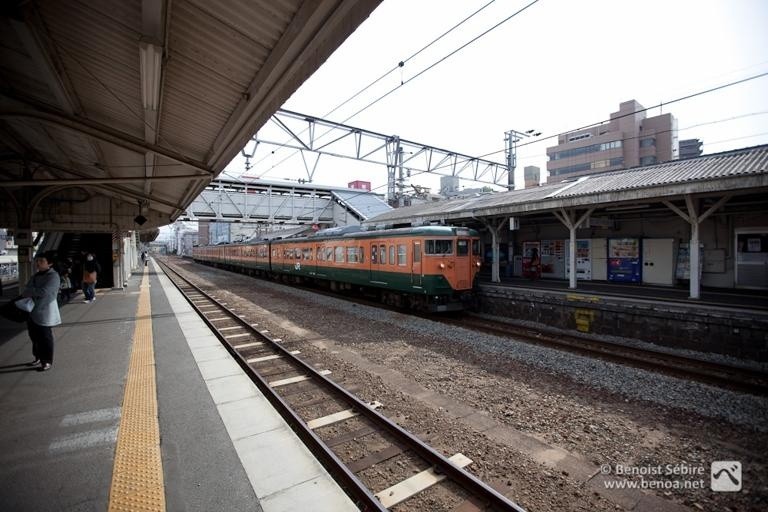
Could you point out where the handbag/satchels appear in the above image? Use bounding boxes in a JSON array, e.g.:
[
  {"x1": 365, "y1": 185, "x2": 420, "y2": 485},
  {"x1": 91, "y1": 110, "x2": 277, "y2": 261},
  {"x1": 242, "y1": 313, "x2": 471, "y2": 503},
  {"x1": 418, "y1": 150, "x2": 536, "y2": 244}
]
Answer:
[
  {"x1": 0, "y1": 296, "x2": 29, "y2": 323},
  {"x1": 83, "y1": 271, "x2": 97, "y2": 283},
  {"x1": 14, "y1": 298, "x2": 35, "y2": 313}
]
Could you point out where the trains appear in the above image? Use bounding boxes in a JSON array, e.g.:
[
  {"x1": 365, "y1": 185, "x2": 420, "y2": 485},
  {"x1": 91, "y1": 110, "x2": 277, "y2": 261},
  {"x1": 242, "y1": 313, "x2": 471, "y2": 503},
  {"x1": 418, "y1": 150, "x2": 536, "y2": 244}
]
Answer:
[
  {"x1": 191, "y1": 222, "x2": 483, "y2": 312},
  {"x1": 0, "y1": 256, "x2": 18, "y2": 286}
]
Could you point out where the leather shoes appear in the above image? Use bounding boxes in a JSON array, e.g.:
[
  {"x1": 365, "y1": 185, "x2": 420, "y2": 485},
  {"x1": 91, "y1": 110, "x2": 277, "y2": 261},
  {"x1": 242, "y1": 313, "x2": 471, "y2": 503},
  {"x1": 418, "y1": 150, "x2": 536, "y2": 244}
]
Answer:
[
  {"x1": 42, "y1": 362, "x2": 51, "y2": 370},
  {"x1": 32, "y1": 358, "x2": 41, "y2": 366}
]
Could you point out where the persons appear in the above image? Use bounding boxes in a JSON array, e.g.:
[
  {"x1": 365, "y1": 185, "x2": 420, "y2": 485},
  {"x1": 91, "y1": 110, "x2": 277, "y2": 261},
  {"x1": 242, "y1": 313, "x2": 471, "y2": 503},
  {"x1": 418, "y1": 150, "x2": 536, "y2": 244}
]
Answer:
[
  {"x1": 21, "y1": 253, "x2": 63, "y2": 371},
  {"x1": 44, "y1": 252, "x2": 103, "y2": 304},
  {"x1": 144, "y1": 250, "x2": 149, "y2": 265},
  {"x1": 530, "y1": 248, "x2": 539, "y2": 283}
]
